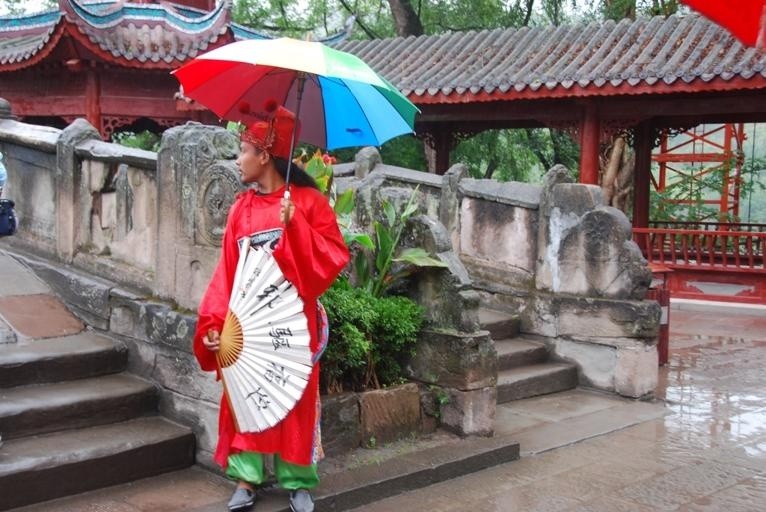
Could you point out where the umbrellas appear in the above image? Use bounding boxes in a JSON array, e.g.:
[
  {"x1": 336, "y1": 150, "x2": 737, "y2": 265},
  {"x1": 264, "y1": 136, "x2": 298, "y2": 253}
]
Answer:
[{"x1": 168, "y1": 29, "x2": 424, "y2": 226}]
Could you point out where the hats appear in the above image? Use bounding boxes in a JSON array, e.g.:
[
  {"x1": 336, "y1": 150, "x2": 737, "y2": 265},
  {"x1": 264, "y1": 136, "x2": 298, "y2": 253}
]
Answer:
[{"x1": 238, "y1": 96, "x2": 302, "y2": 160}]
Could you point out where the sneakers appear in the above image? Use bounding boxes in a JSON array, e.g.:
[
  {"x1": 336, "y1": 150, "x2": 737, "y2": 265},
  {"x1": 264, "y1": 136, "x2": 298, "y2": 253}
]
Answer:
[
  {"x1": 228, "y1": 487, "x2": 256, "y2": 510},
  {"x1": 289, "y1": 488, "x2": 315, "y2": 512}
]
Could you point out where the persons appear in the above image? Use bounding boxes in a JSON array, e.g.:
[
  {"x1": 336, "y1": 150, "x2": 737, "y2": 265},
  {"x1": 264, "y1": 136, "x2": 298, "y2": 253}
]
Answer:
[{"x1": 189, "y1": 123, "x2": 354, "y2": 512}]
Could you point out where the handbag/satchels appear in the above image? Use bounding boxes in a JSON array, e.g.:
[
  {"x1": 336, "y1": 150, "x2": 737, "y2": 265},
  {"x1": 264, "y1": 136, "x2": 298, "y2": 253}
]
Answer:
[{"x1": 0, "y1": 199, "x2": 17, "y2": 237}]
